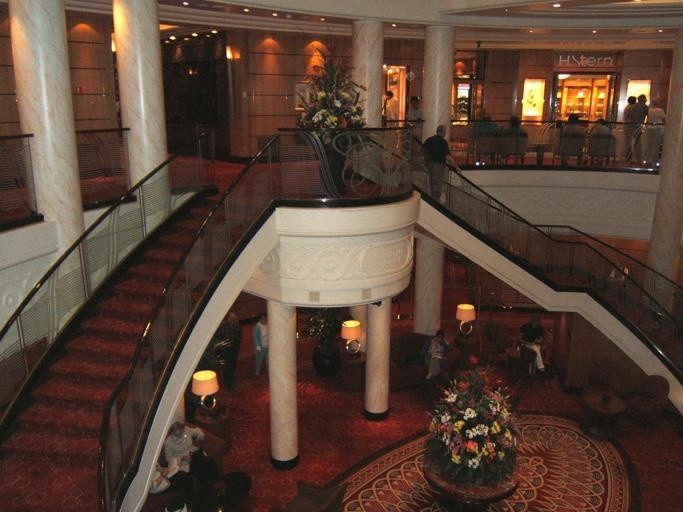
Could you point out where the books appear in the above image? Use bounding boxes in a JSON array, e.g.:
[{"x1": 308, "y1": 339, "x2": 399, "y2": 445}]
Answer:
[{"x1": 167, "y1": 460, "x2": 179, "y2": 479}]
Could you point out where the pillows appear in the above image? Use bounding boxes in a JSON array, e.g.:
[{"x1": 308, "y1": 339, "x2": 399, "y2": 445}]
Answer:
[
  {"x1": 482, "y1": 323, "x2": 512, "y2": 346},
  {"x1": 399, "y1": 338, "x2": 422, "y2": 365}
]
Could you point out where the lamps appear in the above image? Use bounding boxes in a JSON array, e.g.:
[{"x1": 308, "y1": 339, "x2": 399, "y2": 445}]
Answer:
[
  {"x1": 455, "y1": 304, "x2": 477, "y2": 336},
  {"x1": 190, "y1": 370, "x2": 218, "y2": 410},
  {"x1": 341, "y1": 320, "x2": 362, "y2": 360}
]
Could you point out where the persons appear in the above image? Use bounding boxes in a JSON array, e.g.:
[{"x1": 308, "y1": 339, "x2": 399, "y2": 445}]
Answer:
[
  {"x1": 642, "y1": 96, "x2": 665, "y2": 163},
  {"x1": 588, "y1": 119, "x2": 614, "y2": 166},
  {"x1": 518, "y1": 317, "x2": 545, "y2": 377},
  {"x1": 151, "y1": 461, "x2": 194, "y2": 498},
  {"x1": 406, "y1": 97, "x2": 422, "y2": 153},
  {"x1": 499, "y1": 117, "x2": 526, "y2": 165},
  {"x1": 381, "y1": 90, "x2": 395, "y2": 141},
  {"x1": 621, "y1": 97, "x2": 636, "y2": 163},
  {"x1": 253, "y1": 313, "x2": 269, "y2": 378},
  {"x1": 424, "y1": 125, "x2": 461, "y2": 203},
  {"x1": 422, "y1": 329, "x2": 449, "y2": 382},
  {"x1": 609, "y1": 262, "x2": 629, "y2": 281},
  {"x1": 558, "y1": 115, "x2": 585, "y2": 167},
  {"x1": 214, "y1": 311, "x2": 243, "y2": 390},
  {"x1": 626, "y1": 95, "x2": 649, "y2": 162},
  {"x1": 474, "y1": 115, "x2": 504, "y2": 163},
  {"x1": 165, "y1": 422, "x2": 217, "y2": 485}
]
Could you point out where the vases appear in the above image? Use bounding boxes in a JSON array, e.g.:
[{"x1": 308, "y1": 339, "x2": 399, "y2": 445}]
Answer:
[
  {"x1": 422, "y1": 453, "x2": 520, "y2": 512},
  {"x1": 308, "y1": 138, "x2": 348, "y2": 196}
]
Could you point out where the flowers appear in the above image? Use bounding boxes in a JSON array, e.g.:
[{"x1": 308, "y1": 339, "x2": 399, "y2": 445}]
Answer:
[
  {"x1": 427, "y1": 362, "x2": 519, "y2": 485},
  {"x1": 292, "y1": 45, "x2": 367, "y2": 144}
]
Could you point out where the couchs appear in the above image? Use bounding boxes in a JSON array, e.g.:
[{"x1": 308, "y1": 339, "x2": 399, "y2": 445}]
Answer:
[
  {"x1": 143, "y1": 422, "x2": 227, "y2": 512},
  {"x1": 389, "y1": 331, "x2": 460, "y2": 392},
  {"x1": 479, "y1": 318, "x2": 553, "y2": 365},
  {"x1": 624, "y1": 374, "x2": 670, "y2": 433}
]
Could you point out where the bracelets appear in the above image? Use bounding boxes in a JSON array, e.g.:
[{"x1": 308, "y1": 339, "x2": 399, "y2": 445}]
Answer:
[{"x1": 456, "y1": 167, "x2": 461, "y2": 173}]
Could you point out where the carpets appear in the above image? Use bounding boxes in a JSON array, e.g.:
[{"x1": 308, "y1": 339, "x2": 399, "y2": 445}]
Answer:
[{"x1": 322, "y1": 410, "x2": 643, "y2": 512}]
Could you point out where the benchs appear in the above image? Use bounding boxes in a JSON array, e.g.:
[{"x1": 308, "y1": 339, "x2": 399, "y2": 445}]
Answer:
[
  {"x1": 466, "y1": 153, "x2": 526, "y2": 165},
  {"x1": 552, "y1": 153, "x2": 616, "y2": 168}
]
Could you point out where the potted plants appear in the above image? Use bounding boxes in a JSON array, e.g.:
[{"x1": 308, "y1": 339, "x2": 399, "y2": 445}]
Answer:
[
  {"x1": 164, "y1": 497, "x2": 188, "y2": 512},
  {"x1": 196, "y1": 335, "x2": 232, "y2": 392},
  {"x1": 296, "y1": 308, "x2": 354, "y2": 377}
]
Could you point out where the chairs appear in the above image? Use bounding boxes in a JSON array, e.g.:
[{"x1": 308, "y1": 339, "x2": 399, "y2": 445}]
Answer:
[{"x1": 270, "y1": 479, "x2": 346, "y2": 512}]
